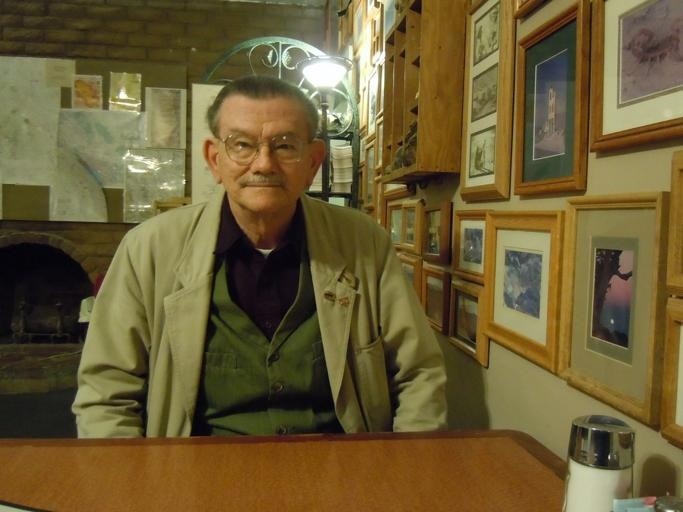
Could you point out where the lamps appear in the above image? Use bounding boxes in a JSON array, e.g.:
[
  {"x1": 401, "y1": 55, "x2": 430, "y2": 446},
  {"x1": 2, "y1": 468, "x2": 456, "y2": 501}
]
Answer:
[{"x1": 294, "y1": 56, "x2": 354, "y2": 200}]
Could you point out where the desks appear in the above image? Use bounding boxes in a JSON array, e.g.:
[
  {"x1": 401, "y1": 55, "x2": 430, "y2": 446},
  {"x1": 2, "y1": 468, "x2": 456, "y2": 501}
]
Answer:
[{"x1": 1, "y1": 426, "x2": 569, "y2": 510}]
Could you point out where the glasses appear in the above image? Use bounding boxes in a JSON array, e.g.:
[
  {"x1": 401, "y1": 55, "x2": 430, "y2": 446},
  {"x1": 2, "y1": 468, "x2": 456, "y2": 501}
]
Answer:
[{"x1": 216, "y1": 132, "x2": 311, "y2": 164}]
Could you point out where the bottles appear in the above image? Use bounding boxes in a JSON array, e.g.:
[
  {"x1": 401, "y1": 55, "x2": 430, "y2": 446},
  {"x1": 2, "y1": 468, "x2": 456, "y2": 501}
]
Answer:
[{"x1": 558, "y1": 412, "x2": 635, "y2": 512}]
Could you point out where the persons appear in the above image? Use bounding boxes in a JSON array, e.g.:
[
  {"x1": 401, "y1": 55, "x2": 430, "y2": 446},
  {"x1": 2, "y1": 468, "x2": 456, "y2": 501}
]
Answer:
[{"x1": 68, "y1": 76, "x2": 448, "y2": 440}]
